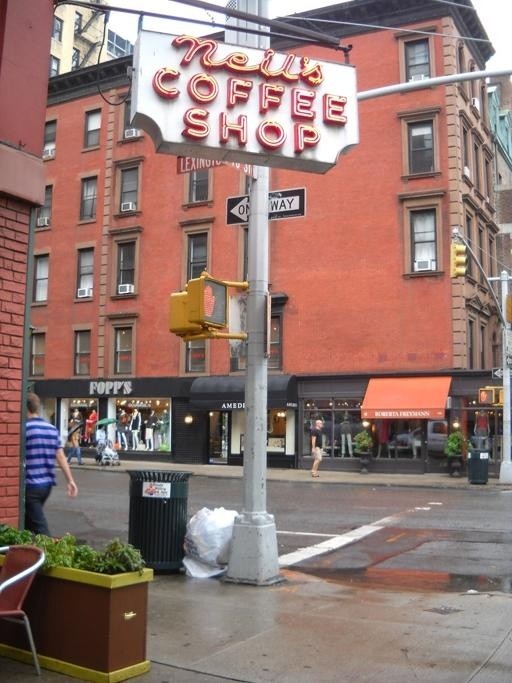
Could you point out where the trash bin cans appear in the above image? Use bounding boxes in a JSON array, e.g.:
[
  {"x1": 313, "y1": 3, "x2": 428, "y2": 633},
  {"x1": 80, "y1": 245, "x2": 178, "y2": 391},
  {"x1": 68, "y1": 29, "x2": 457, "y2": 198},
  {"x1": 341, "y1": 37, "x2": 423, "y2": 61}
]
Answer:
[
  {"x1": 467, "y1": 449, "x2": 490, "y2": 484},
  {"x1": 125, "y1": 470, "x2": 194, "y2": 575}
]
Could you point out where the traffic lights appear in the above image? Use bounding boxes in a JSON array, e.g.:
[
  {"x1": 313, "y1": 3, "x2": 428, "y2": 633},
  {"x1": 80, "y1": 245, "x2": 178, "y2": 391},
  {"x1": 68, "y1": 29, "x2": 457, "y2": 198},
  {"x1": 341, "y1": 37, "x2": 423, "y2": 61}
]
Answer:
[
  {"x1": 187, "y1": 276, "x2": 229, "y2": 329},
  {"x1": 450, "y1": 243, "x2": 468, "y2": 279}
]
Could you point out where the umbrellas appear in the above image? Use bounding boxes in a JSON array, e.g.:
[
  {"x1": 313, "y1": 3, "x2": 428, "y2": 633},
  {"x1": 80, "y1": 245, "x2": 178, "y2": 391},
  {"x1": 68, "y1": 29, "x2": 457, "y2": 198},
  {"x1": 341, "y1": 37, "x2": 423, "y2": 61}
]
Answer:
[
  {"x1": 96, "y1": 416, "x2": 119, "y2": 433},
  {"x1": 68, "y1": 422, "x2": 85, "y2": 439}
]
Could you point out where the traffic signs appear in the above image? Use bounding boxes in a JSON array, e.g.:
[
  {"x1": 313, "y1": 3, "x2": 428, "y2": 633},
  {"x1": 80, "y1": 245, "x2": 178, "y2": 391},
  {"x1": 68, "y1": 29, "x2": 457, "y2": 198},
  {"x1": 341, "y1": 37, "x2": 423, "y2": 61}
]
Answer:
[
  {"x1": 492, "y1": 368, "x2": 512, "y2": 379},
  {"x1": 225, "y1": 186, "x2": 306, "y2": 226}
]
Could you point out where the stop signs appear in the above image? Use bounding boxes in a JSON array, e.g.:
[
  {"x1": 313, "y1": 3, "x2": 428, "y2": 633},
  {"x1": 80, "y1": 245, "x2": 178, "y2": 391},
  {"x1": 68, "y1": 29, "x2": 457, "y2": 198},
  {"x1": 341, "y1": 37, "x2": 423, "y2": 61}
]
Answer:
[{"x1": 478, "y1": 389, "x2": 495, "y2": 404}]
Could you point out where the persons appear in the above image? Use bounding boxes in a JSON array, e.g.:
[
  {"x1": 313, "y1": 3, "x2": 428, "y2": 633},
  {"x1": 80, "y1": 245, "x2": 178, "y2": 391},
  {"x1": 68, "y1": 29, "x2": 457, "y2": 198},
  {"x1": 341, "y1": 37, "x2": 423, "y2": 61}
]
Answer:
[
  {"x1": 144, "y1": 409, "x2": 158, "y2": 450},
  {"x1": 86, "y1": 408, "x2": 97, "y2": 447},
  {"x1": 25, "y1": 391, "x2": 78, "y2": 537},
  {"x1": 67, "y1": 426, "x2": 86, "y2": 465},
  {"x1": 405, "y1": 418, "x2": 422, "y2": 458},
  {"x1": 473, "y1": 409, "x2": 490, "y2": 448},
  {"x1": 117, "y1": 409, "x2": 130, "y2": 451},
  {"x1": 339, "y1": 410, "x2": 354, "y2": 457},
  {"x1": 310, "y1": 405, "x2": 328, "y2": 453},
  {"x1": 157, "y1": 408, "x2": 171, "y2": 451},
  {"x1": 130, "y1": 407, "x2": 142, "y2": 449},
  {"x1": 96, "y1": 424, "x2": 106, "y2": 464},
  {"x1": 375, "y1": 419, "x2": 391, "y2": 457},
  {"x1": 310, "y1": 419, "x2": 322, "y2": 477},
  {"x1": 104, "y1": 444, "x2": 117, "y2": 461},
  {"x1": 70, "y1": 408, "x2": 84, "y2": 445}
]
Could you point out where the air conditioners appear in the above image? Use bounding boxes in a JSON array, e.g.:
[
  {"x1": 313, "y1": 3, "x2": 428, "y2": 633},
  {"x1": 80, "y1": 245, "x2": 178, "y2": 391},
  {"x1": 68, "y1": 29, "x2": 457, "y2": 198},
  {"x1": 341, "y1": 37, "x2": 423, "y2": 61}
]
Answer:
[
  {"x1": 124, "y1": 129, "x2": 137, "y2": 139},
  {"x1": 78, "y1": 288, "x2": 93, "y2": 298},
  {"x1": 43, "y1": 148, "x2": 56, "y2": 158},
  {"x1": 121, "y1": 202, "x2": 136, "y2": 212},
  {"x1": 413, "y1": 259, "x2": 431, "y2": 271},
  {"x1": 411, "y1": 74, "x2": 424, "y2": 82},
  {"x1": 464, "y1": 167, "x2": 470, "y2": 177},
  {"x1": 118, "y1": 283, "x2": 134, "y2": 294},
  {"x1": 36, "y1": 217, "x2": 50, "y2": 227},
  {"x1": 471, "y1": 98, "x2": 480, "y2": 111}
]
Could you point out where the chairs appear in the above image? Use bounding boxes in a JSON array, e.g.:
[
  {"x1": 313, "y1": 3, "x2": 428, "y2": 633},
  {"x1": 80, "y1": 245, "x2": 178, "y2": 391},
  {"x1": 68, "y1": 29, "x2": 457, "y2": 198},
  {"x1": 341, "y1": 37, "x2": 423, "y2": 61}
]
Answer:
[{"x1": 0, "y1": 543, "x2": 45, "y2": 677}]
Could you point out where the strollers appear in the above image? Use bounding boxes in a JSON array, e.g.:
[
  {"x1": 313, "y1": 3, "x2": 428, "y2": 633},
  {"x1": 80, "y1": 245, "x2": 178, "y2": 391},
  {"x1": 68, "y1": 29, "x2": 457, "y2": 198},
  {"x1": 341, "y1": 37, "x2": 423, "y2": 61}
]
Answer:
[{"x1": 99, "y1": 439, "x2": 120, "y2": 466}]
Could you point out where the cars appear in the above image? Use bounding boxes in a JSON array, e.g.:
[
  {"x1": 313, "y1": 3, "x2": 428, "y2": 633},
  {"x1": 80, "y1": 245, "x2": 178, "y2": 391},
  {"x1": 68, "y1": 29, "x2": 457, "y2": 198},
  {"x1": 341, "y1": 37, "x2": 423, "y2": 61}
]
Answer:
[{"x1": 398, "y1": 421, "x2": 449, "y2": 451}]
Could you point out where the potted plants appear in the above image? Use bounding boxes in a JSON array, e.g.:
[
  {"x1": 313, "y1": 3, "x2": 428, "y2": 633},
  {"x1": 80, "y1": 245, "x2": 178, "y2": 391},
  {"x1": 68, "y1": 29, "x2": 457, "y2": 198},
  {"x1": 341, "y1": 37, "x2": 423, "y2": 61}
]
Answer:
[
  {"x1": 443, "y1": 431, "x2": 474, "y2": 477},
  {"x1": 0, "y1": 524, "x2": 154, "y2": 683},
  {"x1": 353, "y1": 429, "x2": 374, "y2": 473}
]
